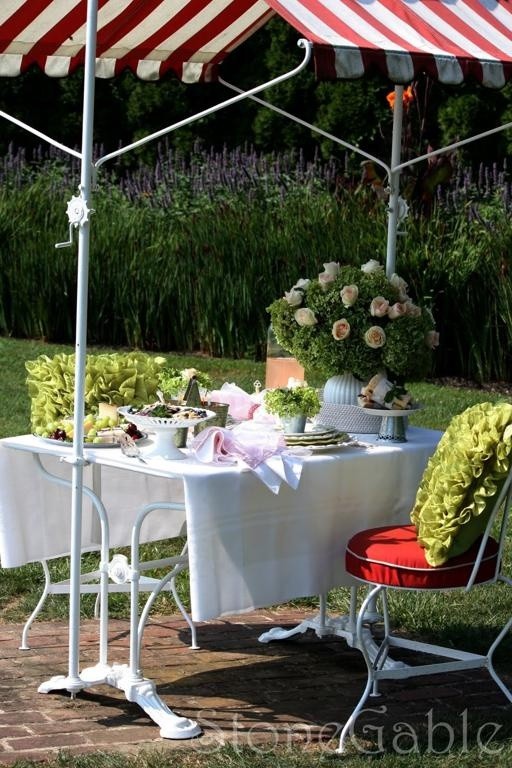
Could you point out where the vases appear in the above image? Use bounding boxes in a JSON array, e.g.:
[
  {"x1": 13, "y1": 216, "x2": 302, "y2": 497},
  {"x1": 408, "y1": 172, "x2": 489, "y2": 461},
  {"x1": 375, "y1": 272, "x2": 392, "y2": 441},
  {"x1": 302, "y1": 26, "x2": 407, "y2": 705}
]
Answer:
[{"x1": 322, "y1": 369, "x2": 369, "y2": 406}]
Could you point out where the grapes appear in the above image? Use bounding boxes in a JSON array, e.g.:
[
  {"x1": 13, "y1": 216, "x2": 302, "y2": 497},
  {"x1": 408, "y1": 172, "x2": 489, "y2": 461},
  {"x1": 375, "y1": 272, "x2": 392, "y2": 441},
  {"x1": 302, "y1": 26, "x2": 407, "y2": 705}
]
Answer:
[{"x1": 35, "y1": 414, "x2": 142, "y2": 444}]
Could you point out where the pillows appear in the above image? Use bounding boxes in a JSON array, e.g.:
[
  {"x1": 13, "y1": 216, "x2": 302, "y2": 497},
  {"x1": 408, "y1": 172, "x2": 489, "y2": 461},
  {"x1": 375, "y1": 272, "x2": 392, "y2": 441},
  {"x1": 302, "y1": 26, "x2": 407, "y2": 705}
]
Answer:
[
  {"x1": 24, "y1": 348, "x2": 163, "y2": 427},
  {"x1": 410, "y1": 401, "x2": 512, "y2": 567}
]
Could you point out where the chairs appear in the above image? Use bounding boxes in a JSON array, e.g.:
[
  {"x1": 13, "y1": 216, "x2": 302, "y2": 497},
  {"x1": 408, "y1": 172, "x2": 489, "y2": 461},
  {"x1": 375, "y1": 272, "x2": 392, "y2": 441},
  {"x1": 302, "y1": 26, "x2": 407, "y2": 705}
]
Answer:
[{"x1": 329, "y1": 447, "x2": 511, "y2": 761}]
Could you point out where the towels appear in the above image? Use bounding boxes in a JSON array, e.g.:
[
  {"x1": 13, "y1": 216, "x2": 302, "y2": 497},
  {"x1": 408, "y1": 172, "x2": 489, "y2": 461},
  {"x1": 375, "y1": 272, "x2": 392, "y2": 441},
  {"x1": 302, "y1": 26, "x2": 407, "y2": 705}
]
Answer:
[{"x1": 186, "y1": 421, "x2": 315, "y2": 499}]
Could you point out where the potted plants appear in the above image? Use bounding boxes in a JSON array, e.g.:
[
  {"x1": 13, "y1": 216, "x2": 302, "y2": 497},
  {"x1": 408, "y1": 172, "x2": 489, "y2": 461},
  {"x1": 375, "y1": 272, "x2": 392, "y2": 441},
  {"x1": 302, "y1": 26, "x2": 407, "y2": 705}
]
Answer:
[{"x1": 263, "y1": 385, "x2": 322, "y2": 434}]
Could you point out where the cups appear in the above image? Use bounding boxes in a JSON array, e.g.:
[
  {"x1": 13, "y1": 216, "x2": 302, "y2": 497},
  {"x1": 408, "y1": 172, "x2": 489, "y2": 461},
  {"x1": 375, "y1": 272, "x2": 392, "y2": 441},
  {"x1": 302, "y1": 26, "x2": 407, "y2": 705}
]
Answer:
[{"x1": 163, "y1": 399, "x2": 230, "y2": 448}]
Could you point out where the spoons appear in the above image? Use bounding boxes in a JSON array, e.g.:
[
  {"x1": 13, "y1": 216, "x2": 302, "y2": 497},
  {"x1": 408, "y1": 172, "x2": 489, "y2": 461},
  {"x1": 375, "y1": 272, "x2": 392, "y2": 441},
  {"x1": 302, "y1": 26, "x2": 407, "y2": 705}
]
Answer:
[{"x1": 119, "y1": 433, "x2": 148, "y2": 466}]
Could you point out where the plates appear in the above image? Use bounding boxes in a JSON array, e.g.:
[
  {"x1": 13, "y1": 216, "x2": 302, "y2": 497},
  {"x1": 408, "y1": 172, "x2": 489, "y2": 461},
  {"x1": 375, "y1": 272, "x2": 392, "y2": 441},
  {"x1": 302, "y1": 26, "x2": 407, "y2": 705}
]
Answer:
[{"x1": 35, "y1": 430, "x2": 148, "y2": 448}]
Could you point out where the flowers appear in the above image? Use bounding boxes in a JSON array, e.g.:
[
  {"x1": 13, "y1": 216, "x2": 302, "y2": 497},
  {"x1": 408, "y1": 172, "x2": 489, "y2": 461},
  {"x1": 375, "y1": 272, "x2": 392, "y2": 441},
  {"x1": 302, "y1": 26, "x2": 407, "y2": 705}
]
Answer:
[{"x1": 262, "y1": 257, "x2": 444, "y2": 384}]
